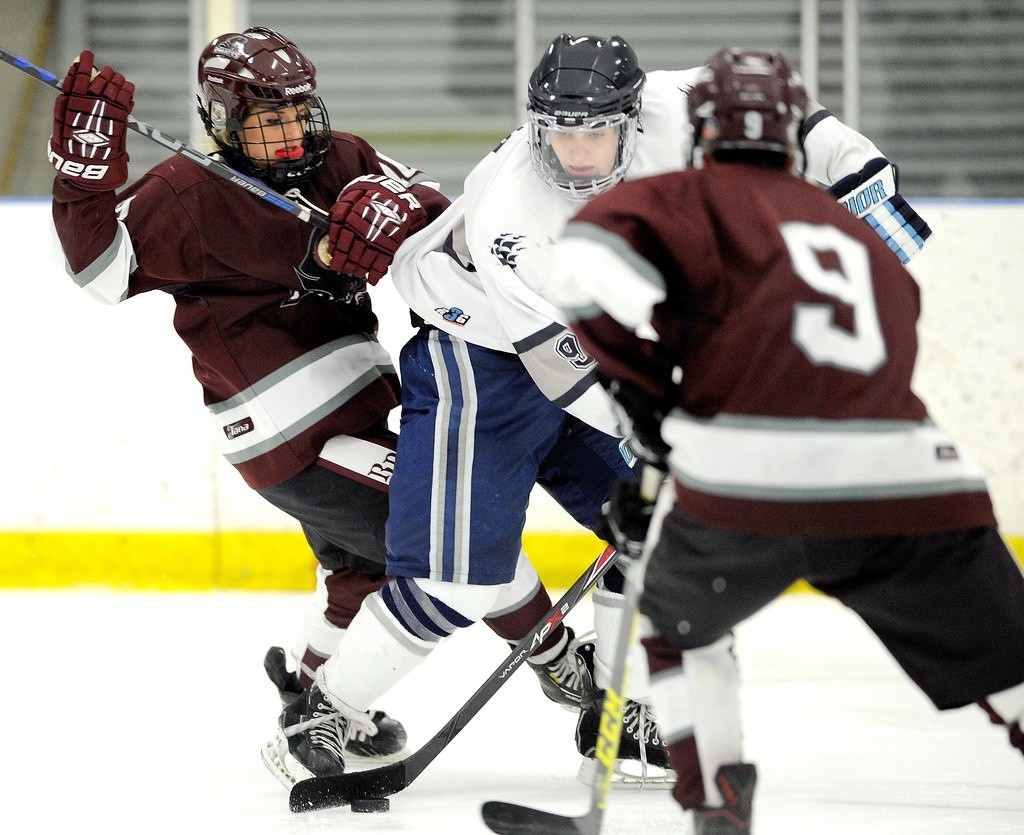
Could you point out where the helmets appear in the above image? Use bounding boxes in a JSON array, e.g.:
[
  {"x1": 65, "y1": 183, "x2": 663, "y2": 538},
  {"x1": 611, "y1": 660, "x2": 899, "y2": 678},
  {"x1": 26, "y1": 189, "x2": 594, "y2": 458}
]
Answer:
[
  {"x1": 528, "y1": 34, "x2": 647, "y2": 199},
  {"x1": 197, "y1": 27, "x2": 333, "y2": 184},
  {"x1": 688, "y1": 47, "x2": 806, "y2": 160}
]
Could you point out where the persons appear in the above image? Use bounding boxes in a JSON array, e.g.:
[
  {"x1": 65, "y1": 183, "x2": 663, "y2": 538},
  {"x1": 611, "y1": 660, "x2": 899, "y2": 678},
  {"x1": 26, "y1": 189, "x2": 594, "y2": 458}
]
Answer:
[
  {"x1": 549, "y1": 49, "x2": 1024, "y2": 835},
  {"x1": 47, "y1": 26, "x2": 596, "y2": 756},
  {"x1": 266, "y1": 32, "x2": 933, "y2": 785}
]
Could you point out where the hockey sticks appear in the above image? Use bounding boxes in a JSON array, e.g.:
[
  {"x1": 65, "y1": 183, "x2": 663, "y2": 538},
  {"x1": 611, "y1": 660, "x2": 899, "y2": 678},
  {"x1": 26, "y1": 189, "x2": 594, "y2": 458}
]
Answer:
[
  {"x1": 479, "y1": 462, "x2": 664, "y2": 835},
  {"x1": 286, "y1": 541, "x2": 623, "y2": 817},
  {"x1": 1, "y1": 49, "x2": 332, "y2": 232}
]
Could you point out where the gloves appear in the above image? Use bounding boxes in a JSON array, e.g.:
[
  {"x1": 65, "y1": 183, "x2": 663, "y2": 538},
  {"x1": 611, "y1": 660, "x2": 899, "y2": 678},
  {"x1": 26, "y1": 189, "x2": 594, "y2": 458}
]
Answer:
[
  {"x1": 596, "y1": 484, "x2": 656, "y2": 563},
  {"x1": 325, "y1": 173, "x2": 425, "y2": 285},
  {"x1": 48, "y1": 49, "x2": 134, "y2": 190}
]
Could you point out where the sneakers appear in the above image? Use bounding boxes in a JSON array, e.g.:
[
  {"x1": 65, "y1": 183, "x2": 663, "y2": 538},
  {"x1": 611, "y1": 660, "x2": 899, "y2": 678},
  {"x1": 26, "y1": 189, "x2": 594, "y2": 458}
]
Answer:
[
  {"x1": 263, "y1": 645, "x2": 411, "y2": 764},
  {"x1": 261, "y1": 665, "x2": 352, "y2": 793},
  {"x1": 509, "y1": 626, "x2": 595, "y2": 708},
  {"x1": 571, "y1": 638, "x2": 680, "y2": 794}
]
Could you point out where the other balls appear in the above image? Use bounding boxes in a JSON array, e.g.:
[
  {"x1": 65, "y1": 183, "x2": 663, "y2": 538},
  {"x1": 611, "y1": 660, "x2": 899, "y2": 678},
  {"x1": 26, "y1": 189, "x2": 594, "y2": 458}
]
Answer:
[{"x1": 348, "y1": 797, "x2": 392, "y2": 815}]
